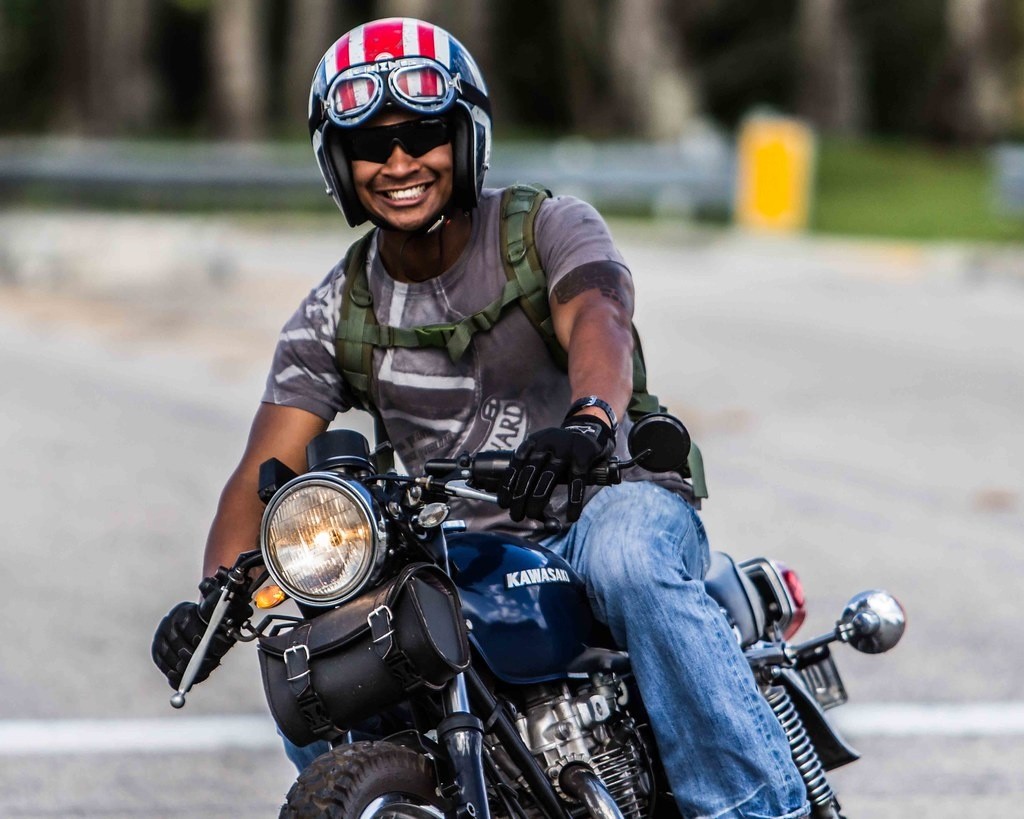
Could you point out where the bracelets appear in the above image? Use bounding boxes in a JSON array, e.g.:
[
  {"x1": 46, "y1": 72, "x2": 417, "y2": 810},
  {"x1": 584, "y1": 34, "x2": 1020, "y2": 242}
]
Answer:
[{"x1": 560, "y1": 394, "x2": 620, "y2": 432}]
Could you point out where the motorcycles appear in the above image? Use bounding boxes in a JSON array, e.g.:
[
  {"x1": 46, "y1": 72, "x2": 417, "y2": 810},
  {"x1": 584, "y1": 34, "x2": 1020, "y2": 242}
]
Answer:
[{"x1": 169, "y1": 429, "x2": 908, "y2": 819}]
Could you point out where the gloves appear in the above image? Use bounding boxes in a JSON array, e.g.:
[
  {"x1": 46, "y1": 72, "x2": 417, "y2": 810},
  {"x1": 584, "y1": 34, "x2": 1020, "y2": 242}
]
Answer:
[
  {"x1": 151, "y1": 601, "x2": 228, "y2": 691},
  {"x1": 498, "y1": 413, "x2": 616, "y2": 525}
]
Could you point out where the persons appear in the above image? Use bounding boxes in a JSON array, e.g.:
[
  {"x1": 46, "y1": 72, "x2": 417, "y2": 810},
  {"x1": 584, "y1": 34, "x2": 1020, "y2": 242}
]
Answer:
[{"x1": 148, "y1": 16, "x2": 812, "y2": 819}]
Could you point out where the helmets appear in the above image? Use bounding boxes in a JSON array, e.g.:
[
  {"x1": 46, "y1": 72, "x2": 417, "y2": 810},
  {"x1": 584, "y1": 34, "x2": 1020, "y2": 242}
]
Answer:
[{"x1": 307, "y1": 16, "x2": 491, "y2": 228}]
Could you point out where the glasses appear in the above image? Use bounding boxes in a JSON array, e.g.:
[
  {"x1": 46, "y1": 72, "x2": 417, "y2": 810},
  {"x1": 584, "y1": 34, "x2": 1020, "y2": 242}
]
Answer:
[
  {"x1": 343, "y1": 117, "x2": 450, "y2": 163},
  {"x1": 323, "y1": 55, "x2": 460, "y2": 126}
]
